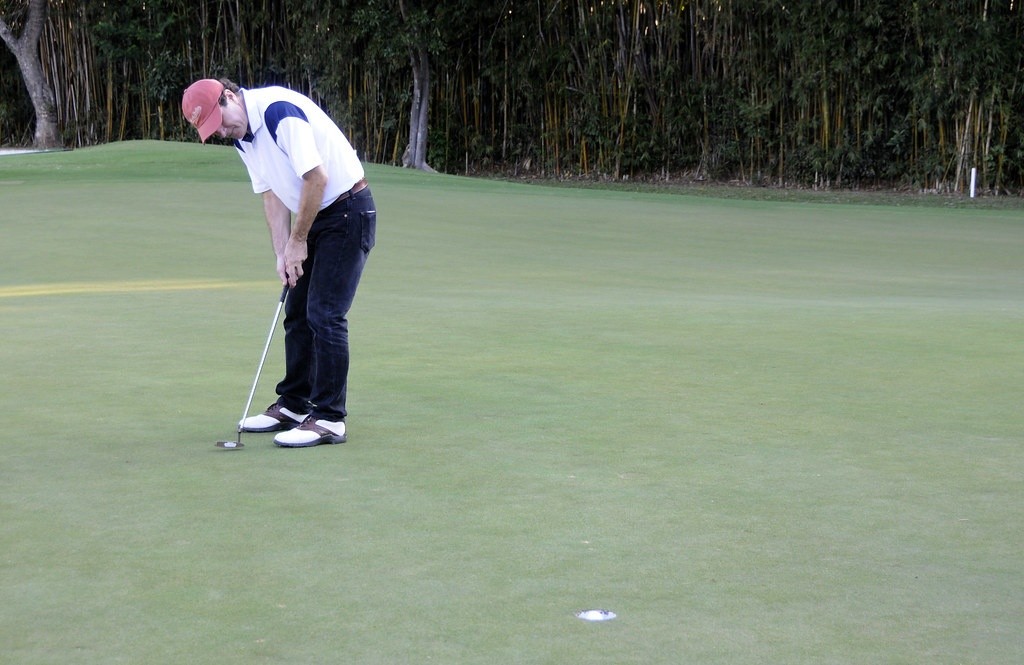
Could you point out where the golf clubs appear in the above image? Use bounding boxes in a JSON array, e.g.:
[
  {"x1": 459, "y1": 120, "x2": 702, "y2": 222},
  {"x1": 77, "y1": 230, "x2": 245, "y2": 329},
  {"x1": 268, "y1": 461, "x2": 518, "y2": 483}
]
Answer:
[{"x1": 215, "y1": 270, "x2": 292, "y2": 449}]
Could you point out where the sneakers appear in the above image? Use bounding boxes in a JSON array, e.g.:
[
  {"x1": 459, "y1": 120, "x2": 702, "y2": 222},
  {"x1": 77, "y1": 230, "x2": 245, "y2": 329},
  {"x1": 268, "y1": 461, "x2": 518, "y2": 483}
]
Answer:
[
  {"x1": 239, "y1": 402, "x2": 312, "y2": 432},
  {"x1": 273, "y1": 414, "x2": 346, "y2": 447}
]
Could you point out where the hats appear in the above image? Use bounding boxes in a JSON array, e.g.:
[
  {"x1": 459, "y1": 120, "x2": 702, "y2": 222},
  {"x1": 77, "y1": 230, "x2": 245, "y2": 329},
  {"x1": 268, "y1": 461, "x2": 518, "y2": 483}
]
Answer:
[{"x1": 182, "y1": 79, "x2": 224, "y2": 144}]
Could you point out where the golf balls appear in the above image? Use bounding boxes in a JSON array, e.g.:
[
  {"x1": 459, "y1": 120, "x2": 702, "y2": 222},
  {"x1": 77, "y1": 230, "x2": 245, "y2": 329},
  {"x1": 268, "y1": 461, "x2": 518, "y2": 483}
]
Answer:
[{"x1": 223, "y1": 441, "x2": 237, "y2": 448}]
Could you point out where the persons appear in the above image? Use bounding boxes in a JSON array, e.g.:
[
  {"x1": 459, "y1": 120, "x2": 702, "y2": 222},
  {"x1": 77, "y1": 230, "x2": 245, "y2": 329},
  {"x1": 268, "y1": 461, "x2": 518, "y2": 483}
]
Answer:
[{"x1": 182, "y1": 79, "x2": 377, "y2": 447}]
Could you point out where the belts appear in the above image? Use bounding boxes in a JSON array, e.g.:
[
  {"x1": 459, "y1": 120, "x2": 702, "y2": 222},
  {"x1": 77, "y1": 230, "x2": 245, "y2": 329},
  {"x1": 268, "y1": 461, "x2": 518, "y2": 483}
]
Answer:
[{"x1": 331, "y1": 178, "x2": 368, "y2": 205}]
